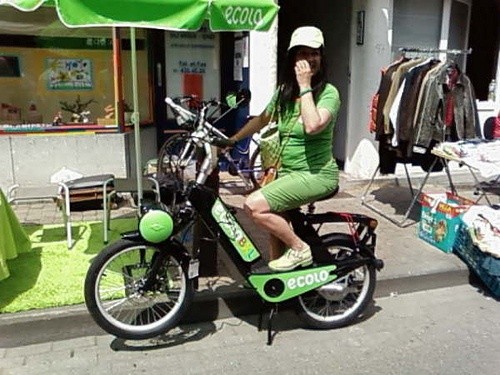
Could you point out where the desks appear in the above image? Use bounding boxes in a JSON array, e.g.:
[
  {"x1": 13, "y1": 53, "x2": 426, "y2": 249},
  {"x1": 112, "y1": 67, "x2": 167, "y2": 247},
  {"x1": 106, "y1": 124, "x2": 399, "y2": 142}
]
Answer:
[{"x1": 427, "y1": 146, "x2": 500, "y2": 204}]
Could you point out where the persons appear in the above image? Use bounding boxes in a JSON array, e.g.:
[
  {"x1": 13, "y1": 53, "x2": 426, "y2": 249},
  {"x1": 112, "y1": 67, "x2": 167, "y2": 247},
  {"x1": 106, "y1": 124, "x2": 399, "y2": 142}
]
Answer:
[{"x1": 218, "y1": 26, "x2": 340, "y2": 272}]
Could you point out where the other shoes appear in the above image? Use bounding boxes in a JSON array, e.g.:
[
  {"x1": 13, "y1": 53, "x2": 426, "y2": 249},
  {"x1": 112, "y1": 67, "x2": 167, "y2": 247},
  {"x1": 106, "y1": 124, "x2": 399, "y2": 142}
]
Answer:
[{"x1": 268, "y1": 244, "x2": 313, "y2": 272}]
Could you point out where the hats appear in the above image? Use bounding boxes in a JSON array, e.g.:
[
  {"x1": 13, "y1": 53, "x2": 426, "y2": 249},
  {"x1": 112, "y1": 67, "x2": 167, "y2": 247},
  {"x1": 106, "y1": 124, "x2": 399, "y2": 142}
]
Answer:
[{"x1": 288, "y1": 26, "x2": 325, "y2": 51}]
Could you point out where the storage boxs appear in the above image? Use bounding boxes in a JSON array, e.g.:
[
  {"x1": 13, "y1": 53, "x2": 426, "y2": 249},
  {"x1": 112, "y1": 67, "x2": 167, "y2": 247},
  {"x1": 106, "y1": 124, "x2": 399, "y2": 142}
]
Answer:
[{"x1": 419, "y1": 193, "x2": 478, "y2": 253}]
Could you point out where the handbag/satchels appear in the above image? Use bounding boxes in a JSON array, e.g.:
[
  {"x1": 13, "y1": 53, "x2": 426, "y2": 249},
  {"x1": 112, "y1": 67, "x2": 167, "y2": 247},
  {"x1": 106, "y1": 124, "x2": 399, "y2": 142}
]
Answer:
[
  {"x1": 258, "y1": 166, "x2": 278, "y2": 189},
  {"x1": 260, "y1": 126, "x2": 280, "y2": 170}
]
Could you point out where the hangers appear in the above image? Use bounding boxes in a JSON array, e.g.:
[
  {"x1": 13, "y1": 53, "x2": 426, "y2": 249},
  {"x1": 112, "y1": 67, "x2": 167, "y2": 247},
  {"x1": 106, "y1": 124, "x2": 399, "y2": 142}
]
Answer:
[{"x1": 398, "y1": 46, "x2": 457, "y2": 62}]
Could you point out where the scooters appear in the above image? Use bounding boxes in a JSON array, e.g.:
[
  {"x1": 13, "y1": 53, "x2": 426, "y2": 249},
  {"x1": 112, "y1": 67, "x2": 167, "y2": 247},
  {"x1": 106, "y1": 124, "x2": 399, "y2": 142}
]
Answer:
[{"x1": 84, "y1": 98, "x2": 384, "y2": 347}]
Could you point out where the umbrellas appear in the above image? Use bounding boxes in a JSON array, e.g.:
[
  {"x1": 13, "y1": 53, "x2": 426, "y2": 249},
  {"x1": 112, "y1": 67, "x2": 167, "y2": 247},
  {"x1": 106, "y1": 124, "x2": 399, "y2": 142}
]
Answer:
[
  {"x1": 0, "y1": 190, "x2": 30, "y2": 282},
  {"x1": 0, "y1": 0, "x2": 281, "y2": 216}
]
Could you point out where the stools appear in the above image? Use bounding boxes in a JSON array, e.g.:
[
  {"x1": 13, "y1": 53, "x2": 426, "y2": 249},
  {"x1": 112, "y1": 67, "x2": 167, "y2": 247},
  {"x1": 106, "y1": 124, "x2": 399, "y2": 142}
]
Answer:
[
  {"x1": 7, "y1": 180, "x2": 73, "y2": 249},
  {"x1": 102, "y1": 177, "x2": 162, "y2": 243}
]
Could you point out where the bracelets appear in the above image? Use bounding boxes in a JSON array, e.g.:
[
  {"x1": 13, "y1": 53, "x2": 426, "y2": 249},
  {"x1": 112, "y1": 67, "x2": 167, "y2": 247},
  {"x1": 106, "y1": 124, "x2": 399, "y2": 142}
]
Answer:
[{"x1": 298, "y1": 88, "x2": 313, "y2": 96}]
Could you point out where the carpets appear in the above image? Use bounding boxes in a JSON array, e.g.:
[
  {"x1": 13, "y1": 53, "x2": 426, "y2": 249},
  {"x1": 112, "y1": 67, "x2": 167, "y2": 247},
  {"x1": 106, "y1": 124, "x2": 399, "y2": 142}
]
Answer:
[{"x1": 0, "y1": 217, "x2": 174, "y2": 313}]
